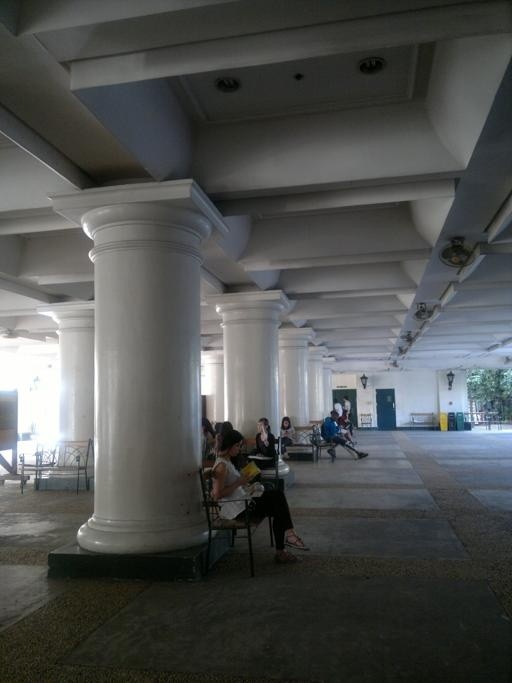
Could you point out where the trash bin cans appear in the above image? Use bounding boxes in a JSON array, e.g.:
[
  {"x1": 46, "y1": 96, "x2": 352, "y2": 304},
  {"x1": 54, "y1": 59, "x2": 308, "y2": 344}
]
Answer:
[{"x1": 439, "y1": 412, "x2": 464, "y2": 431}]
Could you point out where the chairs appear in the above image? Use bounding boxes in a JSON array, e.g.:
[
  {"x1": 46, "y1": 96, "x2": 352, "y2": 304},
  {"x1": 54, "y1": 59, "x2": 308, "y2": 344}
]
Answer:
[{"x1": 361, "y1": 414, "x2": 373, "y2": 431}]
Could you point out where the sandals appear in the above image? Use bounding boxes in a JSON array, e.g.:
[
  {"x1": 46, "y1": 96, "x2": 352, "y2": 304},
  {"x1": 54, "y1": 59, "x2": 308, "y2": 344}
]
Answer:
[
  {"x1": 275, "y1": 551, "x2": 303, "y2": 564},
  {"x1": 286, "y1": 535, "x2": 310, "y2": 551}
]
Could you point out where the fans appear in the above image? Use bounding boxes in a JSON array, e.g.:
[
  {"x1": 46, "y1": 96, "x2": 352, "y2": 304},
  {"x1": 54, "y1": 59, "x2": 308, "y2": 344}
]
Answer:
[
  {"x1": 413, "y1": 303, "x2": 433, "y2": 321},
  {"x1": 439, "y1": 237, "x2": 476, "y2": 267},
  {"x1": 400, "y1": 330, "x2": 417, "y2": 344}
]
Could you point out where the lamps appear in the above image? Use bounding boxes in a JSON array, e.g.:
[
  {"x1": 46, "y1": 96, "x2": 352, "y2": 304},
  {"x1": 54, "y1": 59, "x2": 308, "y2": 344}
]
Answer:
[
  {"x1": 360, "y1": 373, "x2": 369, "y2": 390},
  {"x1": 446, "y1": 370, "x2": 455, "y2": 390}
]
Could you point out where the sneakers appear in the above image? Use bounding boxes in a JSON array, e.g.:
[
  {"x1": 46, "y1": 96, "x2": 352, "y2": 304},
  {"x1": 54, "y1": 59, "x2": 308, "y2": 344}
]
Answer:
[
  {"x1": 328, "y1": 449, "x2": 336, "y2": 458},
  {"x1": 359, "y1": 453, "x2": 368, "y2": 459}
]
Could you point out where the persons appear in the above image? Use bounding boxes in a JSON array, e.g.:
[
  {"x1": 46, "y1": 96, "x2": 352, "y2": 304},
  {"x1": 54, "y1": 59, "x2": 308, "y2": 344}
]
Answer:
[
  {"x1": 254, "y1": 417, "x2": 278, "y2": 463},
  {"x1": 211, "y1": 428, "x2": 312, "y2": 564},
  {"x1": 342, "y1": 395, "x2": 352, "y2": 417},
  {"x1": 277, "y1": 415, "x2": 297, "y2": 459},
  {"x1": 342, "y1": 409, "x2": 354, "y2": 436},
  {"x1": 333, "y1": 398, "x2": 344, "y2": 416},
  {"x1": 330, "y1": 409, "x2": 359, "y2": 446},
  {"x1": 320, "y1": 410, "x2": 368, "y2": 460},
  {"x1": 200, "y1": 417, "x2": 249, "y2": 471}
]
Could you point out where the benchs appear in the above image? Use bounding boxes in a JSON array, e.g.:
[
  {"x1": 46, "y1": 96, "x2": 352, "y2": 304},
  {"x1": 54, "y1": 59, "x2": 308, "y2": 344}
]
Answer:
[
  {"x1": 284, "y1": 419, "x2": 339, "y2": 462},
  {"x1": 408, "y1": 412, "x2": 439, "y2": 432},
  {"x1": 196, "y1": 436, "x2": 281, "y2": 578},
  {"x1": 464, "y1": 411, "x2": 503, "y2": 430},
  {"x1": 17, "y1": 438, "x2": 92, "y2": 496}
]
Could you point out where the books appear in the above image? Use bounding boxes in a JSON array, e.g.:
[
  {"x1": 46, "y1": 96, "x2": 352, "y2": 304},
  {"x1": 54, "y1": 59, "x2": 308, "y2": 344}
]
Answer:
[
  {"x1": 247, "y1": 454, "x2": 273, "y2": 460},
  {"x1": 239, "y1": 459, "x2": 262, "y2": 483}
]
